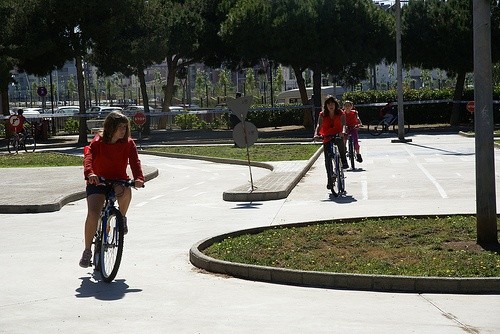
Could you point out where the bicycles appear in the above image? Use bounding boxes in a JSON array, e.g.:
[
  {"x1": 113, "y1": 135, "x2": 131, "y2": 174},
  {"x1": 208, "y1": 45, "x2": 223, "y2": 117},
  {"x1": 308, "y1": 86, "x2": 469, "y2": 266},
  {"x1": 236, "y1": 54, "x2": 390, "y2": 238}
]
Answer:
[
  {"x1": 6, "y1": 122, "x2": 36, "y2": 153},
  {"x1": 368, "y1": 106, "x2": 410, "y2": 136},
  {"x1": 312, "y1": 125, "x2": 364, "y2": 198},
  {"x1": 84, "y1": 177, "x2": 146, "y2": 283}
]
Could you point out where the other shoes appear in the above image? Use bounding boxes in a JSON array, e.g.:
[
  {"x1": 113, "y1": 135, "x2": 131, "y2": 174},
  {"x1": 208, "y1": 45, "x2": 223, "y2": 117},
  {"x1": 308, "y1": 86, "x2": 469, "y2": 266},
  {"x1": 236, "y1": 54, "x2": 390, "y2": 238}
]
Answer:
[
  {"x1": 343, "y1": 160, "x2": 349, "y2": 169},
  {"x1": 327, "y1": 178, "x2": 335, "y2": 189},
  {"x1": 384, "y1": 122, "x2": 388, "y2": 126},
  {"x1": 357, "y1": 154, "x2": 363, "y2": 162}
]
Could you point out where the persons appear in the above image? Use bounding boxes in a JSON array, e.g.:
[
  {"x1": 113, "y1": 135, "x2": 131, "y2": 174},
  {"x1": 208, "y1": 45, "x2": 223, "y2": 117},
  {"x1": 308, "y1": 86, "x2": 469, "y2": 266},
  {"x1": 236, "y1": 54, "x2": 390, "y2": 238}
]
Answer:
[
  {"x1": 314, "y1": 96, "x2": 349, "y2": 189},
  {"x1": 13, "y1": 109, "x2": 31, "y2": 148},
  {"x1": 77, "y1": 111, "x2": 144, "y2": 267},
  {"x1": 343, "y1": 101, "x2": 362, "y2": 162},
  {"x1": 380, "y1": 100, "x2": 393, "y2": 132}
]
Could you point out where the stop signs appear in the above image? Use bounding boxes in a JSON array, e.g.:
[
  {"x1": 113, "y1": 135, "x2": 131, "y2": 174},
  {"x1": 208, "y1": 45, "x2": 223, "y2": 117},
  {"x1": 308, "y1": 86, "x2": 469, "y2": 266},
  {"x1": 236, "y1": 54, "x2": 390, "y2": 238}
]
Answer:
[
  {"x1": 133, "y1": 112, "x2": 146, "y2": 125},
  {"x1": 467, "y1": 101, "x2": 475, "y2": 112}
]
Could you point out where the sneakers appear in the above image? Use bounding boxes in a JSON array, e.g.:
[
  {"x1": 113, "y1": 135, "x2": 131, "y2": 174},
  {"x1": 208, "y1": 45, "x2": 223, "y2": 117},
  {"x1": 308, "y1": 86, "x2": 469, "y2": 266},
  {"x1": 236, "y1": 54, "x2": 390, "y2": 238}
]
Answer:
[
  {"x1": 122, "y1": 216, "x2": 128, "y2": 234},
  {"x1": 79, "y1": 250, "x2": 92, "y2": 268}
]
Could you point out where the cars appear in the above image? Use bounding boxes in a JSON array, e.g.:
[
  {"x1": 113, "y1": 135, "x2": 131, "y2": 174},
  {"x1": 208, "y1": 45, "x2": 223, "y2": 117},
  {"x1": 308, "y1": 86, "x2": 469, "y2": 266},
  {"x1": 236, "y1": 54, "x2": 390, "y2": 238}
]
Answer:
[{"x1": 45, "y1": 104, "x2": 200, "y2": 113}]
